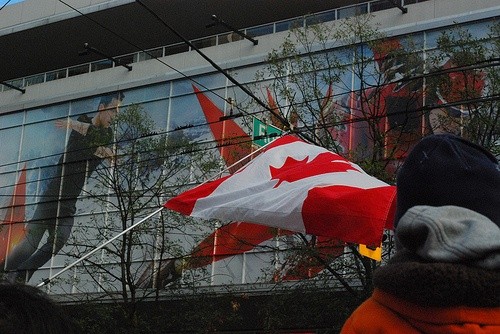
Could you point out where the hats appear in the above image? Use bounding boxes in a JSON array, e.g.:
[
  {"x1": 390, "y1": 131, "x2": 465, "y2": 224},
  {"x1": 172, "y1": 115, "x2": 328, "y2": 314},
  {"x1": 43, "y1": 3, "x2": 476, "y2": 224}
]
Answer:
[{"x1": 395, "y1": 136, "x2": 500, "y2": 230}]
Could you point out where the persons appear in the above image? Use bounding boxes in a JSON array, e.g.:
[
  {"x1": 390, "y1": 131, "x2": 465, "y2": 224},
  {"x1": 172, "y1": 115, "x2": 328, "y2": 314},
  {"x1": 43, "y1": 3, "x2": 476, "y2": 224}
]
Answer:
[
  {"x1": 0, "y1": 90, "x2": 125, "y2": 282},
  {"x1": 340, "y1": 130, "x2": 500, "y2": 334},
  {"x1": 0, "y1": 281, "x2": 84, "y2": 334}
]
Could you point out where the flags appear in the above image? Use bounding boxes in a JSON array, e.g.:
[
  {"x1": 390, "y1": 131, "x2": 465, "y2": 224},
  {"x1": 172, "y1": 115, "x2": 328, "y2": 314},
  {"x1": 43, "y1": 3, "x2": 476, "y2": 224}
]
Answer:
[{"x1": 162, "y1": 133, "x2": 397, "y2": 247}]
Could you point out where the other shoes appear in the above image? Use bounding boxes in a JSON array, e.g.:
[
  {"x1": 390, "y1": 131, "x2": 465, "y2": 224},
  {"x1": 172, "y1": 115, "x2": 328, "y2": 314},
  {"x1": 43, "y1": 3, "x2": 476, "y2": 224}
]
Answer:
[{"x1": 0, "y1": 272, "x2": 24, "y2": 286}]
